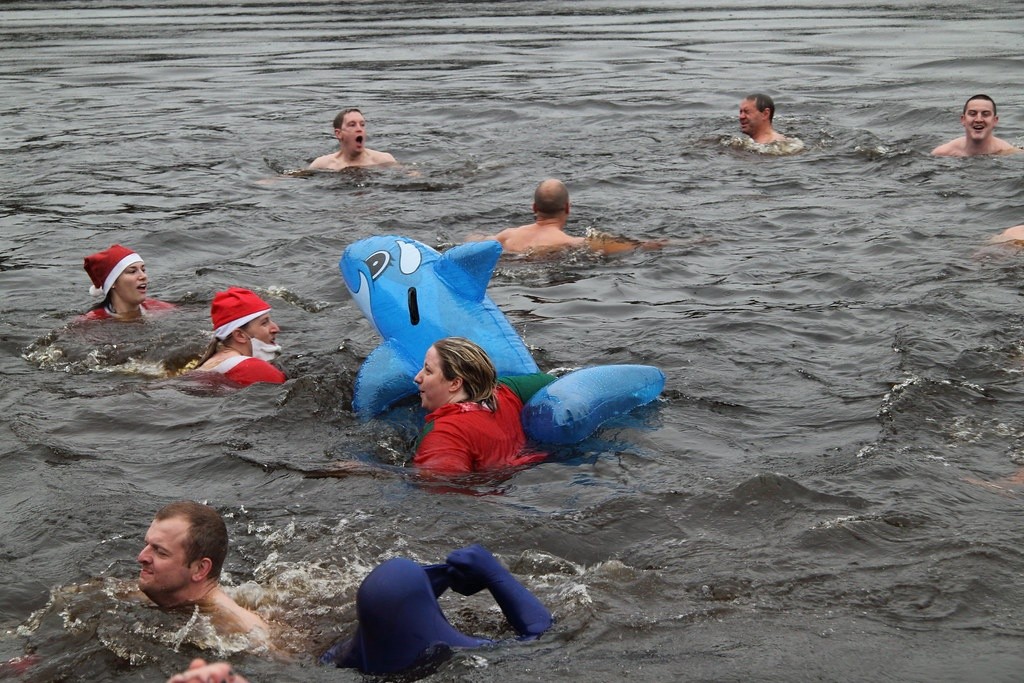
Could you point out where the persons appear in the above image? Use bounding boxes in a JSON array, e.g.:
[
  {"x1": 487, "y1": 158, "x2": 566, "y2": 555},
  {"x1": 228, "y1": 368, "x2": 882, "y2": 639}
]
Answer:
[
  {"x1": 52, "y1": 499, "x2": 300, "y2": 662},
  {"x1": 316, "y1": 545, "x2": 552, "y2": 677},
  {"x1": 160, "y1": 286, "x2": 287, "y2": 391},
  {"x1": 410, "y1": 337, "x2": 558, "y2": 480},
  {"x1": 739, "y1": 93, "x2": 788, "y2": 144},
  {"x1": 254, "y1": 108, "x2": 424, "y2": 184},
  {"x1": 931, "y1": 94, "x2": 1024, "y2": 158},
  {"x1": 76, "y1": 243, "x2": 175, "y2": 323},
  {"x1": 1000, "y1": 225, "x2": 1024, "y2": 259},
  {"x1": 467, "y1": 178, "x2": 670, "y2": 259}
]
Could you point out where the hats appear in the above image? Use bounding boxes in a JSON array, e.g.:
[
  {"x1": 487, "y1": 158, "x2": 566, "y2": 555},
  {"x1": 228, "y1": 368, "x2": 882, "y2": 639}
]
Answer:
[
  {"x1": 83, "y1": 244, "x2": 144, "y2": 297},
  {"x1": 212, "y1": 287, "x2": 272, "y2": 340}
]
